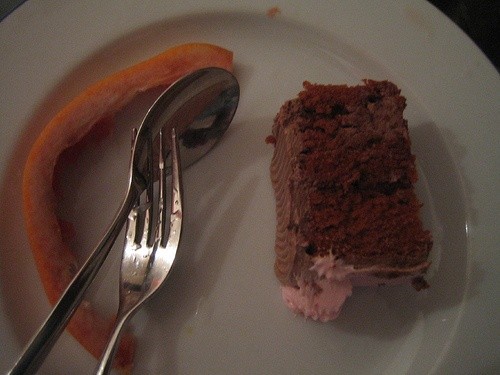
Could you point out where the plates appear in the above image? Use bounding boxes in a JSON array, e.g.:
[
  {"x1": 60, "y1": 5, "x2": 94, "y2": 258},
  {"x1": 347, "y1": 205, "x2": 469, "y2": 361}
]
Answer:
[{"x1": 0, "y1": 0, "x2": 500, "y2": 375}]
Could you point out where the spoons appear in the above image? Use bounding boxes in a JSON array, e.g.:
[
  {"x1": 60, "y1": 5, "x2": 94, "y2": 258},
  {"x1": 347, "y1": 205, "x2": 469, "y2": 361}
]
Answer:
[{"x1": 8, "y1": 65, "x2": 241, "y2": 375}]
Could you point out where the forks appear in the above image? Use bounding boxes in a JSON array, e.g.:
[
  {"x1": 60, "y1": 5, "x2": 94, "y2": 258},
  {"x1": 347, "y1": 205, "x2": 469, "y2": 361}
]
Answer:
[{"x1": 96, "y1": 126, "x2": 184, "y2": 374}]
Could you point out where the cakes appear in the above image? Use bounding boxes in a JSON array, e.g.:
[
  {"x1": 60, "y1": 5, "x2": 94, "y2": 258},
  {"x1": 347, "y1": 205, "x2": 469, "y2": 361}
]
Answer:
[{"x1": 265, "y1": 79, "x2": 433, "y2": 321}]
